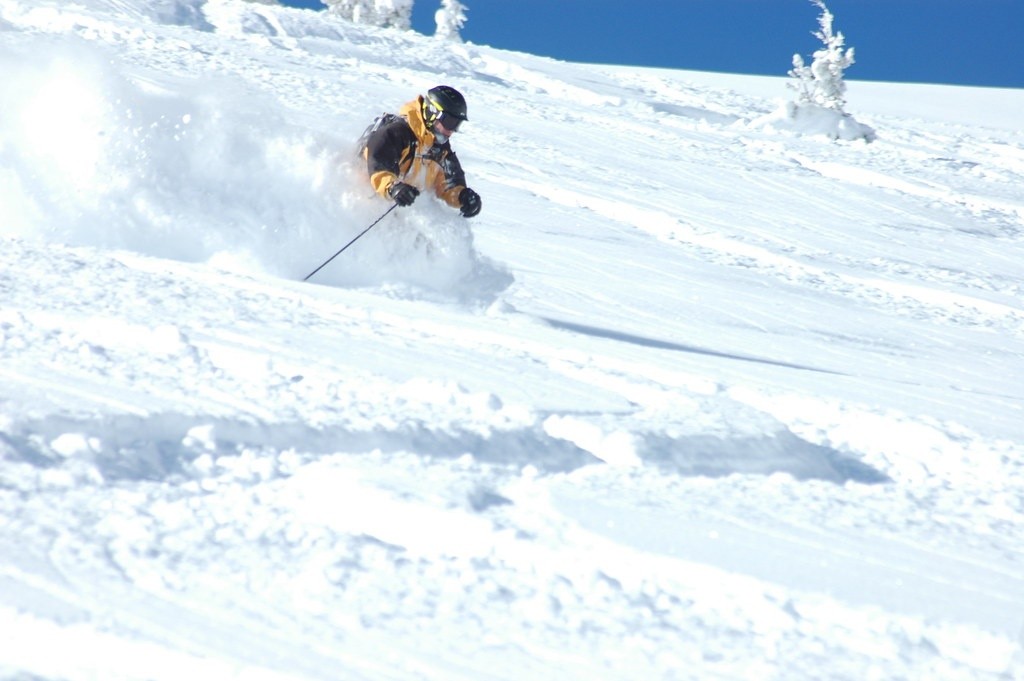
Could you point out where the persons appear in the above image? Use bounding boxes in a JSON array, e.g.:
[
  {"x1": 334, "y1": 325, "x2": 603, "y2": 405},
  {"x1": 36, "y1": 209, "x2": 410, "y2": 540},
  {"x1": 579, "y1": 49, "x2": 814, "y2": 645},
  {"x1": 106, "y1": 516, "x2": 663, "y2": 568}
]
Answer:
[{"x1": 354, "y1": 85, "x2": 482, "y2": 219}]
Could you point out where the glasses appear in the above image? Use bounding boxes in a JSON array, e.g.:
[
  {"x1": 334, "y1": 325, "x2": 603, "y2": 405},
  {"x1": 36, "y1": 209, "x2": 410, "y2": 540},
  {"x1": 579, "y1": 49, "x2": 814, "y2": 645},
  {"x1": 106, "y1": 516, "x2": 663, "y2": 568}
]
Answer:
[{"x1": 436, "y1": 111, "x2": 463, "y2": 132}]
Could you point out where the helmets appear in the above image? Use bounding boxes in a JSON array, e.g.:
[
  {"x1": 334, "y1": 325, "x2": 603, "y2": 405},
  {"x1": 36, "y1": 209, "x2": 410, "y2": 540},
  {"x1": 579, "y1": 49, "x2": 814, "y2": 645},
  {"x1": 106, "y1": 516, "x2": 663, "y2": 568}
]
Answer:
[{"x1": 423, "y1": 85, "x2": 469, "y2": 132}]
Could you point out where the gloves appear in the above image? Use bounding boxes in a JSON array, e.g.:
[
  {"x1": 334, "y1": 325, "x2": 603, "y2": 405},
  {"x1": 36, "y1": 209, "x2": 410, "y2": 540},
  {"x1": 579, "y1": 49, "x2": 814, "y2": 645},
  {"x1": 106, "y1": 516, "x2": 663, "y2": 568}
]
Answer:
[
  {"x1": 389, "y1": 181, "x2": 420, "y2": 207},
  {"x1": 458, "y1": 188, "x2": 481, "y2": 217}
]
